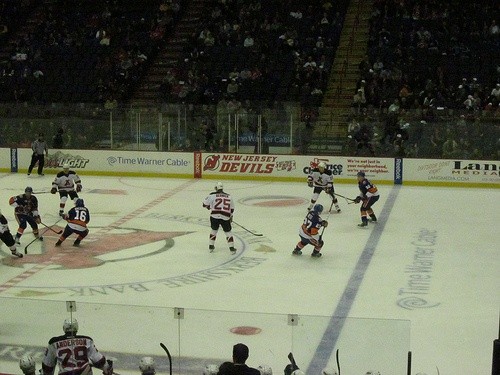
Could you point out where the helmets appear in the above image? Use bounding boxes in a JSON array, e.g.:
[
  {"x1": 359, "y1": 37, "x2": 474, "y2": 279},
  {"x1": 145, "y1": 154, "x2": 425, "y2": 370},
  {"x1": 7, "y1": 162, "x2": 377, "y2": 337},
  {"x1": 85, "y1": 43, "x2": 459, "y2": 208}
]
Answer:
[
  {"x1": 215, "y1": 182, "x2": 223, "y2": 189},
  {"x1": 358, "y1": 171, "x2": 365, "y2": 177},
  {"x1": 139, "y1": 357, "x2": 157, "y2": 374},
  {"x1": 63, "y1": 164, "x2": 71, "y2": 169},
  {"x1": 314, "y1": 204, "x2": 323, "y2": 212},
  {"x1": 75, "y1": 198, "x2": 84, "y2": 204},
  {"x1": 25, "y1": 187, "x2": 34, "y2": 192},
  {"x1": 318, "y1": 162, "x2": 327, "y2": 170},
  {"x1": 63, "y1": 318, "x2": 79, "y2": 333},
  {"x1": 20, "y1": 356, "x2": 36, "y2": 373}
]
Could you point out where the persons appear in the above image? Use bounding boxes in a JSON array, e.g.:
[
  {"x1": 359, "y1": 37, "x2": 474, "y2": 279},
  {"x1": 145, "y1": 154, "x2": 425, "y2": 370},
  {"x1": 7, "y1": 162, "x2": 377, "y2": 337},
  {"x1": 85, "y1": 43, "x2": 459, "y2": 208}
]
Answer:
[
  {"x1": 0, "y1": 0, "x2": 500, "y2": 161},
  {"x1": 20, "y1": 318, "x2": 379, "y2": 375},
  {"x1": 26, "y1": 133, "x2": 48, "y2": 176},
  {"x1": 9, "y1": 187, "x2": 44, "y2": 245},
  {"x1": 51, "y1": 164, "x2": 82, "y2": 217},
  {"x1": 203, "y1": 184, "x2": 235, "y2": 254},
  {"x1": 293, "y1": 204, "x2": 328, "y2": 258},
  {"x1": 306, "y1": 163, "x2": 343, "y2": 214},
  {"x1": 0, "y1": 214, "x2": 24, "y2": 258},
  {"x1": 354, "y1": 171, "x2": 380, "y2": 229},
  {"x1": 55, "y1": 198, "x2": 90, "y2": 248}
]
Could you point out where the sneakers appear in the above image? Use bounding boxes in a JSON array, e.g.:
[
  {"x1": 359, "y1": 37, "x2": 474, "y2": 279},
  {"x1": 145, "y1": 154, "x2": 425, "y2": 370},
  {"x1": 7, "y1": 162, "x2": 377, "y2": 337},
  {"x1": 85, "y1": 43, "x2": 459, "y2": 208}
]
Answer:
[
  {"x1": 312, "y1": 253, "x2": 322, "y2": 257},
  {"x1": 59, "y1": 210, "x2": 65, "y2": 216},
  {"x1": 209, "y1": 245, "x2": 215, "y2": 252},
  {"x1": 293, "y1": 248, "x2": 302, "y2": 254},
  {"x1": 12, "y1": 236, "x2": 81, "y2": 257},
  {"x1": 337, "y1": 209, "x2": 376, "y2": 227},
  {"x1": 230, "y1": 247, "x2": 236, "y2": 253},
  {"x1": 27, "y1": 172, "x2": 45, "y2": 177}
]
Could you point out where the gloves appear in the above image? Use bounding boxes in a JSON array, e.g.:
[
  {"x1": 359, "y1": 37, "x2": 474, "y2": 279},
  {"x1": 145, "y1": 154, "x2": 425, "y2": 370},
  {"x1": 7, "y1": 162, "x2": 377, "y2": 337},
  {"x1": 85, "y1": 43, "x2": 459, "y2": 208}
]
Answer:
[
  {"x1": 51, "y1": 188, "x2": 57, "y2": 194},
  {"x1": 308, "y1": 181, "x2": 313, "y2": 186},
  {"x1": 103, "y1": 360, "x2": 113, "y2": 375},
  {"x1": 76, "y1": 185, "x2": 82, "y2": 192},
  {"x1": 355, "y1": 197, "x2": 361, "y2": 203},
  {"x1": 317, "y1": 240, "x2": 323, "y2": 248},
  {"x1": 321, "y1": 220, "x2": 327, "y2": 228},
  {"x1": 326, "y1": 186, "x2": 332, "y2": 192},
  {"x1": 34, "y1": 215, "x2": 41, "y2": 224}
]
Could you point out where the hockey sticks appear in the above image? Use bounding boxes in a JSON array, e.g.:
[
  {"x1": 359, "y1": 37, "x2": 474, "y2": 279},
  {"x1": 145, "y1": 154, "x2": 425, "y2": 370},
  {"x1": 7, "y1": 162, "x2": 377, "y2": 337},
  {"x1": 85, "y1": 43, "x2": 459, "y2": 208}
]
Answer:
[
  {"x1": 288, "y1": 352, "x2": 296, "y2": 375},
  {"x1": 232, "y1": 220, "x2": 263, "y2": 236},
  {"x1": 336, "y1": 348, "x2": 340, "y2": 375},
  {"x1": 24, "y1": 217, "x2": 63, "y2": 254},
  {"x1": 160, "y1": 343, "x2": 173, "y2": 375},
  {"x1": 318, "y1": 190, "x2": 335, "y2": 244},
  {"x1": 333, "y1": 193, "x2": 360, "y2": 204},
  {"x1": 31, "y1": 190, "x2": 77, "y2": 194},
  {"x1": 40, "y1": 222, "x2": 62, "y2": 235}
]
